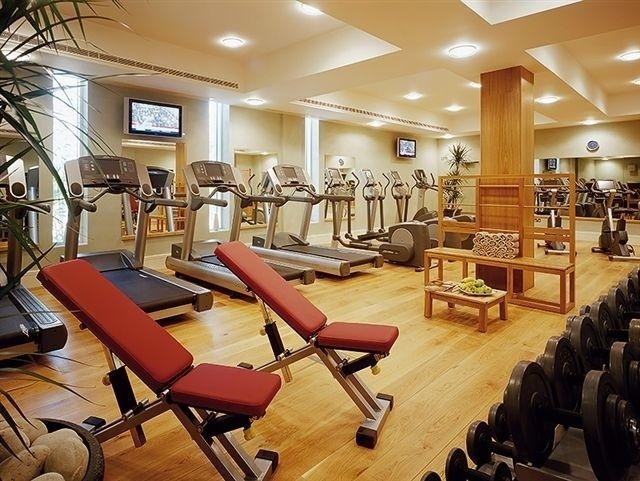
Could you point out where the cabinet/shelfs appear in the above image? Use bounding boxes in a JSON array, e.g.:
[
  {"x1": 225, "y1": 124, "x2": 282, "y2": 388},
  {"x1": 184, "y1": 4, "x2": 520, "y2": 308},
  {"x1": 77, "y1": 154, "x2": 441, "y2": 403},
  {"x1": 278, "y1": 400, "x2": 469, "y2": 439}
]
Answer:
[{"x1": 422, "y1": 172, "x2": 576, "y2": 315}]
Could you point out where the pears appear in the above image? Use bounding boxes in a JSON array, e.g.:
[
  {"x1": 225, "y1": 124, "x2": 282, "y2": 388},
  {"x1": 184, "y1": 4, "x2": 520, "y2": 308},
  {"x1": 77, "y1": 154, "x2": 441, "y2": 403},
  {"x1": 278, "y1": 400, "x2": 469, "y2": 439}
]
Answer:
[{"x1": 472, "y1": 278, "x2": 492, "y2": 294}]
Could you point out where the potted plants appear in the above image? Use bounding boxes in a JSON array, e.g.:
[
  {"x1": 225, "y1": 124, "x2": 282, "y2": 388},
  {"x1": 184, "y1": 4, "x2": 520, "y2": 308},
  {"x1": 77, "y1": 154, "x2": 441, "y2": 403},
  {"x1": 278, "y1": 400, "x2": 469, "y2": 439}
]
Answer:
[{"x1": 440, "y1": 144, "x2": 477, "y2": 219}]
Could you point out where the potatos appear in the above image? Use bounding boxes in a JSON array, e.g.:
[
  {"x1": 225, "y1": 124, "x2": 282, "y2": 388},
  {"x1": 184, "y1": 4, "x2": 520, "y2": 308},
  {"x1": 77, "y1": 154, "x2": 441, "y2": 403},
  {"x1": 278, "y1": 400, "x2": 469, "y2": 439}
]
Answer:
[{"x1": 0, "y1": 418, "x2": 90, "y2": 481}]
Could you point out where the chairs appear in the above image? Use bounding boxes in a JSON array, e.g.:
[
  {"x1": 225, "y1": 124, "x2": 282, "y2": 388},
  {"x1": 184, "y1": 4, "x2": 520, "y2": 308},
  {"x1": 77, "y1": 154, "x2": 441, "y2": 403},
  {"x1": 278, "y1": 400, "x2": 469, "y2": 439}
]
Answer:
[
  {"x1": 214, "y1": 246, "x2": 400, "y2": 449},
  {"x1": 37, "y1": 259, "x2": 281, "y2": 479}
]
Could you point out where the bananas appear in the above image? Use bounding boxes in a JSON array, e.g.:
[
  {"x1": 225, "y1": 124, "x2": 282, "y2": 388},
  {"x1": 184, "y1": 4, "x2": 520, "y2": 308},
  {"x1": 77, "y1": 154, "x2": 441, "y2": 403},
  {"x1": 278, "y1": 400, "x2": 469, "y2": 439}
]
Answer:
[{"x1": 458, "y1": 277, "x2": 474, "y2": 290}]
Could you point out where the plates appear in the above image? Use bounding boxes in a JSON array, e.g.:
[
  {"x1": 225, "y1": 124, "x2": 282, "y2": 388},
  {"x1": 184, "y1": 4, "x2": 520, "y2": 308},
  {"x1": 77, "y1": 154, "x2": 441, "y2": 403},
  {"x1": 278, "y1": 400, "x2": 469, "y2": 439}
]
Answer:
[{"x1": 459, "y1": 286, "x2": 496, "y2": 296}]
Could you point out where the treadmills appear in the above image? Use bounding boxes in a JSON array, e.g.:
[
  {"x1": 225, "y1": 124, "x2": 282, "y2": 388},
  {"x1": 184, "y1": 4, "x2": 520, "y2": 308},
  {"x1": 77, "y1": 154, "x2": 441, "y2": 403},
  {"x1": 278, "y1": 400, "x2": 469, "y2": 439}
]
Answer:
[{"x1": 0, "y1": 154, "x2": 476, "y2": 353}]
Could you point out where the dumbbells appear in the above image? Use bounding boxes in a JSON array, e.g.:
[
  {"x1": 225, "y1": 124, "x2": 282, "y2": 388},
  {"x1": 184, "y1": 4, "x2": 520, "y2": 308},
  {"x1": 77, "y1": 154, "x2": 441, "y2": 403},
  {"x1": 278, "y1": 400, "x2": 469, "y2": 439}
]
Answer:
[{"x1": 420, "y1": 267, "x2": 640, "y2": 481}]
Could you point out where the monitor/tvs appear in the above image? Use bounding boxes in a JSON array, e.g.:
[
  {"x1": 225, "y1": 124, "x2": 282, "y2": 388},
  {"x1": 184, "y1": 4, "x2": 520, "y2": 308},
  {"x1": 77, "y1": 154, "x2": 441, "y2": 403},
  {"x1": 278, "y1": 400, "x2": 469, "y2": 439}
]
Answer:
[
  {"x1": 396, "y1": 137, "x2": 417, "y2": 159},
  {"x1": 129, "y1": 99, "x2": 182, "y2": 137},
  {"x1": 546, "y1": 158, "x2": 557, "y2": 170}
]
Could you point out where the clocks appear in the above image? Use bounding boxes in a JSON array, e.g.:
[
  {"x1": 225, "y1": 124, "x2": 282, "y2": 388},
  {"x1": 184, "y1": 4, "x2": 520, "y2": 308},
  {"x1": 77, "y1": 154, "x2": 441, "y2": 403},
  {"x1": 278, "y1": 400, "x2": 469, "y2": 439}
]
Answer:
[{"x1": 586, "y1": 140, "x2": 600, "y2": 152}]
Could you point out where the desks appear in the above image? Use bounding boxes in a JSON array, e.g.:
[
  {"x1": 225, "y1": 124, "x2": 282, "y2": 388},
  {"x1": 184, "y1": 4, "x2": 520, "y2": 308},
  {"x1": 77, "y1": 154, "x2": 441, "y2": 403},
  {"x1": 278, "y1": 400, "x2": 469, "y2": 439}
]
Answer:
[{"x1": 422, "y1": 281, "x2": 508, "y2": 333}]
